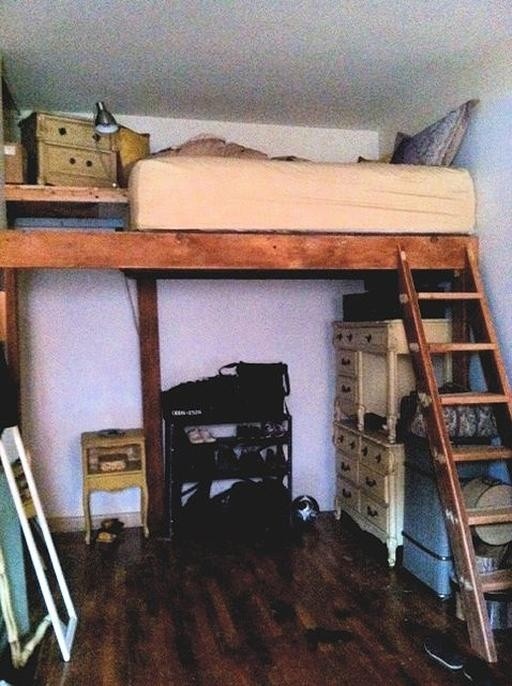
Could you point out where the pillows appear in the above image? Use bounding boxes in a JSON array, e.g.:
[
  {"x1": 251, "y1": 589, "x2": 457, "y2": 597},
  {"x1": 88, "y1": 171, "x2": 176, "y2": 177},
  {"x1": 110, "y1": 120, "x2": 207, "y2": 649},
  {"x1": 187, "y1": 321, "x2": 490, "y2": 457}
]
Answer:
[
  {"x1": 390, "y1": 98, "x2": 480, "y2": 166},
  {"x1": 118, "y1": 125, "x2": 150, "y2": 188}
]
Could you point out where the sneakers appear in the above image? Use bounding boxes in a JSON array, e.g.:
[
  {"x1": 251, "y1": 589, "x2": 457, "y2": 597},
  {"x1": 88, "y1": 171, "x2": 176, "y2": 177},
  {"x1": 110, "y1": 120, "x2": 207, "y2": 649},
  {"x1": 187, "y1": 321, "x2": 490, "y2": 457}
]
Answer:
[
  {"x1": 423, "y1": 632, "x2": 466, "y2": 670},
  {"x1": 463, "y1": 656, "x2": 495, "y2": 683},
  {"x1": 185, "y1": 425, "x2": 204, "y2": 444},
  {"x1": 250, "y1": 425, "x2": 261, "y2": 441},
  {"x1": 262, "y1": 421, "x2": 286, "y2": 438},
  {"x1": 198, "y1": 426, "x2": 216, "y2": 443},
  {"x1": 236, "y1": 425, "x2": 250, "y2": 441}
]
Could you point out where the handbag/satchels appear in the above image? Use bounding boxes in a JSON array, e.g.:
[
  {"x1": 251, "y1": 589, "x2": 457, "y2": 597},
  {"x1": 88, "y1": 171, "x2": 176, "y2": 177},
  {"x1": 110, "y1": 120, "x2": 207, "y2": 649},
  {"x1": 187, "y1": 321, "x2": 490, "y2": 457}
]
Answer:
[
  {"x1": 409, "y1": 382, "x2": 499, "y2": 439},
  {"x1": 218, "y1": 361, "x2": 291, "y2": 425}
]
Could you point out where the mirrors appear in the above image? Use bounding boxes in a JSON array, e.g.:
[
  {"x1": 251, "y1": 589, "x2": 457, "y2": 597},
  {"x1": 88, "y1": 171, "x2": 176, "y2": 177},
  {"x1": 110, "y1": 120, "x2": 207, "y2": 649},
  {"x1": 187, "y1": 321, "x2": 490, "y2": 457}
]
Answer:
[{"x1": 0, "y1": 426, "x2": 77, "y2": 662}]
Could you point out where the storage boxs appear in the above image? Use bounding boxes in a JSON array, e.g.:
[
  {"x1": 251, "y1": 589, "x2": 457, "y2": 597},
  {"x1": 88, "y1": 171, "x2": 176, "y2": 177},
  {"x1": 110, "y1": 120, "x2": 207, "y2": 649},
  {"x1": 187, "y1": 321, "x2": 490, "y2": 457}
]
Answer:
[
  {"x1": 404, "y1": 432, "x2": 490, "y2": 478},
  {"x1": 3, "y1": 141, "x2": 28, "y2": 184},
  {"x1": 0, "y1": 157, "x2": 511, "y2": 668},
  {"x1": 402, "y1": 532, "x2": 455, "y2": 600},
  {"x1": 403, "y1": 462, "x2": 487, "y2": 557}
]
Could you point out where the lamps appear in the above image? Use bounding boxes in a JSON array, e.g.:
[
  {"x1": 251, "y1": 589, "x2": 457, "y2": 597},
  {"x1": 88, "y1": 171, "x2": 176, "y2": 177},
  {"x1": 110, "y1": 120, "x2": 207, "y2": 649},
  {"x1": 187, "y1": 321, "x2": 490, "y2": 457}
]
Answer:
[{"x1": 93, "y1": 101, "x2": 120, "y2": 187}]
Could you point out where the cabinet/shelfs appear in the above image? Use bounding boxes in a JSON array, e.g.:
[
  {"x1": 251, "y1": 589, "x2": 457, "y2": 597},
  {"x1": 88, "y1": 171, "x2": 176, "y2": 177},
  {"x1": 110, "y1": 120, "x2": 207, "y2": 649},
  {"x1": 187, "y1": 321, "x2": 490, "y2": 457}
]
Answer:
[
  {"x1": 17, "y1": 111, "x2": 117, "y2": 186},
  {"x1": 81, "y1": 429, "x2": 150, "y2": 545},
  {"x1": 166, "y1": 414, "x2": 293, "y2": 540},
  {"x1": 333, "y1": 321, "x2": 453, "y2": 568}
]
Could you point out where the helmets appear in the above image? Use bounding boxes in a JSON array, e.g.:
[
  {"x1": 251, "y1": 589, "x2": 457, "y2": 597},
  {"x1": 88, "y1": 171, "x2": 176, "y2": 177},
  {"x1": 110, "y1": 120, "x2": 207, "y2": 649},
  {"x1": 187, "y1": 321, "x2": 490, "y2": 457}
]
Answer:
[{"x1": 294, "y1": 496, "x2": 320, "y2": 520}]
